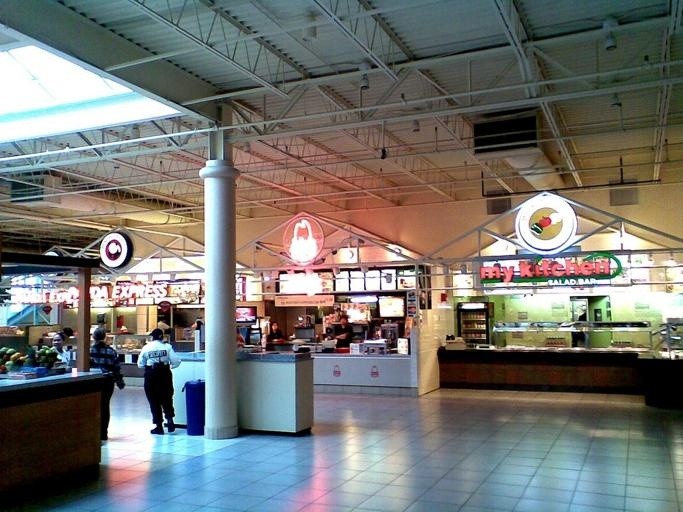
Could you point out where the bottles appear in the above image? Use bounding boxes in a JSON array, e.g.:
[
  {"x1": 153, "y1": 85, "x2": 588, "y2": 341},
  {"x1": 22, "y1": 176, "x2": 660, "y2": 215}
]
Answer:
[
  {"x1": 65, "y1": 345, "x2": 73, "y2": 369},
  {"x1": 675, "y1": 352, "x2": 680, "y2": 360},
  {"x1": 546, "y1": 335, "x2": 566, "y2": 345}
]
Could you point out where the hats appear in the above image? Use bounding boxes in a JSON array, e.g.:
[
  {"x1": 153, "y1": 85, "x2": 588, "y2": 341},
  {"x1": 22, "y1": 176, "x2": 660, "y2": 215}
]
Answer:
[{"x1": 148, "y1": 329, "x2": 163, "y2": 338}]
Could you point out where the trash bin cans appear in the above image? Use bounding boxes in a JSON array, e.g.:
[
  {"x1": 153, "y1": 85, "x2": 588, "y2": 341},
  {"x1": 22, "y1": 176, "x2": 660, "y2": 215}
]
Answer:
[{"x1": 182, "y1": 380, "x2": 205, "y2": 435}]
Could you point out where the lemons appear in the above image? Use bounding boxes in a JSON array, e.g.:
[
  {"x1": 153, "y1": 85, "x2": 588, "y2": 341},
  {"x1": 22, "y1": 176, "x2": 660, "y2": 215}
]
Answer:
[
  {"x1": 40, "y1": 345, "x2": 55, "y2": 356},
  {"x1": 0, "y1": 347, "x2": 35, "y2": 374}
]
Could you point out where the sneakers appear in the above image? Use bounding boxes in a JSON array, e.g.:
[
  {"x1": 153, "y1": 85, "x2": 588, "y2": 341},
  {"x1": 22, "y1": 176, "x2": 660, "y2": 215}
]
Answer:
[
  {"x1": 151, "y1": 427, "x2": 164, "y2": 435},
  {"x1": 167, "y1": 419, "x2": 175, "y2": 432}
]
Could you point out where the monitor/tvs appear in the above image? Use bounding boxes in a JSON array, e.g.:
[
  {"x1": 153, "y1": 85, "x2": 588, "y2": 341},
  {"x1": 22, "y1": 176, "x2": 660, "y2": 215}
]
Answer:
[{"x1": 235, "y1": 306, "x2": 257, "y2": 323}]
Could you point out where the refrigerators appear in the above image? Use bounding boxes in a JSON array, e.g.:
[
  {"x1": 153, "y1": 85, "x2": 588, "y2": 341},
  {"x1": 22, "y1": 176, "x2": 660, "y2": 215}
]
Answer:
[{"x1": 457, "y1": 301, "x2": 492, "y2": 345}]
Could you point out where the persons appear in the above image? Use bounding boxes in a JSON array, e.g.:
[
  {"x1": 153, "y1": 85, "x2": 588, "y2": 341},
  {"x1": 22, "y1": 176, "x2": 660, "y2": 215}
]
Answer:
[
  {"x1": 332, "y1": 314, "x2": 354, "y2": 348},
  {"x1": 49, "y1": 332, "x2": 71, "y2": 364},
  {"x1": 87, "y1": 326, "x2": 127, "y2": 441},
  {"x1": 135, "y1": 328, "x2": 181, "y2": 435},
  {"x1": 266, "y1": 321, "x2": 283, "y2": 341}
]
